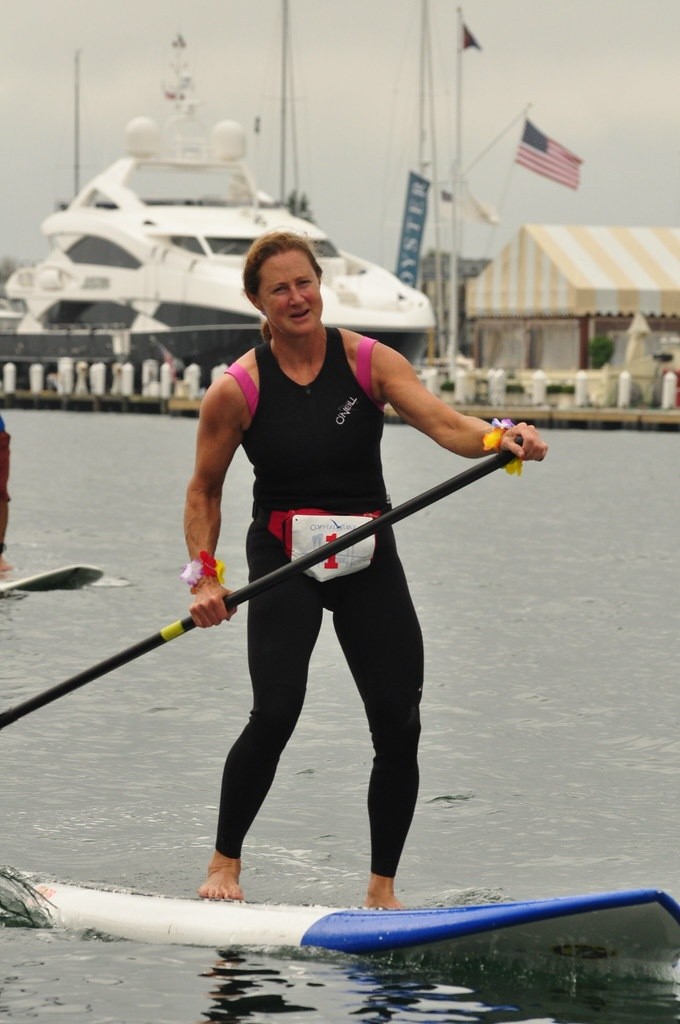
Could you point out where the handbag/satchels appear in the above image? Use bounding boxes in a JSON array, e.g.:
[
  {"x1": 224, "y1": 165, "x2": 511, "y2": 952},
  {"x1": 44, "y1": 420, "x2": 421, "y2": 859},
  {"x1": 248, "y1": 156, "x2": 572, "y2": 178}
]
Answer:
[{"x1": 267, "y1": 509, "x2": 380, "y2": 582}]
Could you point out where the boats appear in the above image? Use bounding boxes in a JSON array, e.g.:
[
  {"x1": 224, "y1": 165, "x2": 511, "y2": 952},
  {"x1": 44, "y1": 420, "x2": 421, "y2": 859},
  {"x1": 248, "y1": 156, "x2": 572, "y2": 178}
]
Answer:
[
  {"x1": 1, "y1": 866, "x2": 680, "y2": 961},
  {"x1": 1, "y1": 34, "x2": 438, "y2": 391}
]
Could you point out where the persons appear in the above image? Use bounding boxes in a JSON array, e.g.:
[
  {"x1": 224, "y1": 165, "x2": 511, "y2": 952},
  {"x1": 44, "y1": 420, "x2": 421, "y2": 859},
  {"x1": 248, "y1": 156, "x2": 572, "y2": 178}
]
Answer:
[{"x1": 180, "y1": 232, "x2": 552, "y2": 911}]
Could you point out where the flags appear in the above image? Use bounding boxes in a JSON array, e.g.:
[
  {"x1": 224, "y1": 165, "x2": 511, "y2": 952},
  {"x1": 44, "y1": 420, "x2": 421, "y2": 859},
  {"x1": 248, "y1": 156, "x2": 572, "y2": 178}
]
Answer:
[
  {"x1": 463, "y1": 182, "x2": 501, "y2": 226},
  {"x1": 461, "y1": 24, "x2": 483, "y2": 52},
  {"x1": 515, "y1": 115, "x2": 584, "y2": 194}
]
